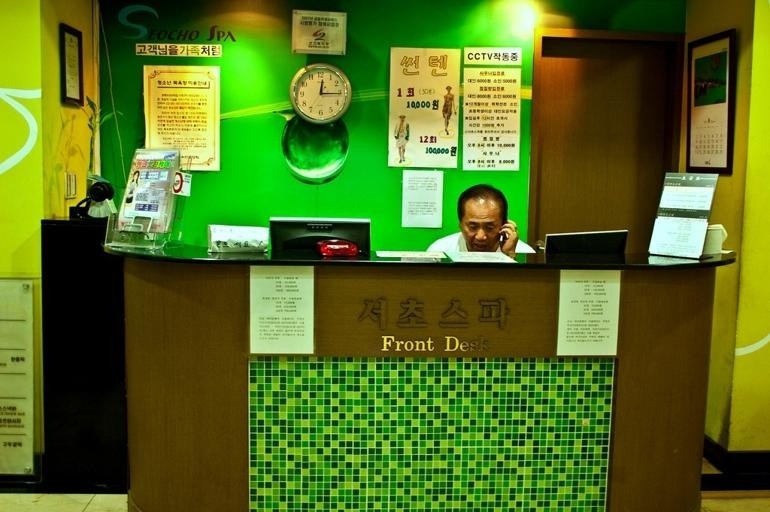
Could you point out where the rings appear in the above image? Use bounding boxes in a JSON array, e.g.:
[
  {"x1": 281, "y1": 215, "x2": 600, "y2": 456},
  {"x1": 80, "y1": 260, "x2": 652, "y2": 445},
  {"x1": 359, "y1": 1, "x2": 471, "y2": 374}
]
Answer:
[{"x1": 512, "y1": 227, "x2": 518, "y2": 232}]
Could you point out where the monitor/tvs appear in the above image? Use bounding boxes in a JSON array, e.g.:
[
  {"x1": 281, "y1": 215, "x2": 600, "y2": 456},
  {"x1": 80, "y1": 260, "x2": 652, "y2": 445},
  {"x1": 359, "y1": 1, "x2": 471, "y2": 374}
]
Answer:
[
  {"x1": 267, "y1": 216, "x2": 371, "y2": 257},
  {"x1": 544, "y1": 229, "x2": 629, "y2": 263}
]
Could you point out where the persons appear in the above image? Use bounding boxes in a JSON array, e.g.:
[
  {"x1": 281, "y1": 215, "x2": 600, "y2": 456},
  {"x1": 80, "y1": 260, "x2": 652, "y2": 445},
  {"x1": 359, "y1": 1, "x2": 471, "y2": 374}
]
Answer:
[
  {"x1": 393, "y1": 114, "x2": 410, "y2": 163},
  {"x1": 426, "y1": 183, "x2": 537, "y2": 253},
  {"x1": 123, "y1": 170, "x2": 140, "y2": 217},
  {"x1": 442, "y1": 85, "x2": 457, "y2": 136}
]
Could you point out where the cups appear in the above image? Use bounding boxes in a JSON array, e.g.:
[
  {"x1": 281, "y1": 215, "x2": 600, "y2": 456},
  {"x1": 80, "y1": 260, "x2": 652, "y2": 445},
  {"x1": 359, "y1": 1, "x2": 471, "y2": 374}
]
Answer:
[{"x1": 702, "y1": 224, "x2": 728, "y2": 254}]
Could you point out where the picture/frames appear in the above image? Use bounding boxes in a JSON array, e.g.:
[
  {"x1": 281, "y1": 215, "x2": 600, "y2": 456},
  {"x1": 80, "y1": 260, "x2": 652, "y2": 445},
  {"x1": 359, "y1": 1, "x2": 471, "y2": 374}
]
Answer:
[
  {"x1": 59, "y1": 22, "x2": 85, "y2": 107},
  {"x1": 685, "y1": 27, "x2": 738, "y2": 176}
]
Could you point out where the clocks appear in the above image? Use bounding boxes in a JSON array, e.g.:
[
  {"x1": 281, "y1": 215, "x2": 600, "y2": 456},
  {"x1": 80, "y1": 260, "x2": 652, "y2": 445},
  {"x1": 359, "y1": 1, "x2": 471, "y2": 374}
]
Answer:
[{"x1": 288, "y1": 62, "x2": 353, "y2": 125}]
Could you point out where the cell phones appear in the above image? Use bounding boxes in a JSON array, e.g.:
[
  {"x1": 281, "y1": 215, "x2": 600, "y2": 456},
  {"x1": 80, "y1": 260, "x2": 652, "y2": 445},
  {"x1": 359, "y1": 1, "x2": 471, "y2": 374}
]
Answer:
[{"x1": 499, "y1": 232, "x2": 505, "y2": 248}]
